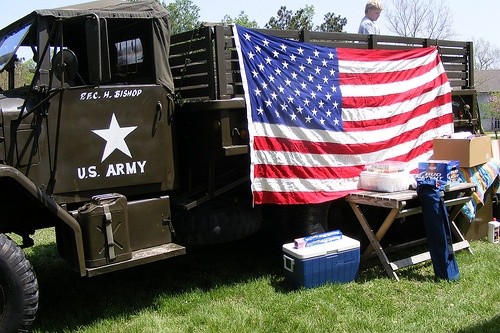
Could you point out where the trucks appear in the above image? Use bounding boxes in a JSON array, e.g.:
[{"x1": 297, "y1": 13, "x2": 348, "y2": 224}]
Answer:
[{"x1": 0, "y1": 1, "x2": 481, "y2": 333}]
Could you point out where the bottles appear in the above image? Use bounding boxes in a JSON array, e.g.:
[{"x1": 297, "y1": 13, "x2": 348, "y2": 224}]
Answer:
[{"x1": 488, "y1": 218, "x2": 500, "y2": 243}]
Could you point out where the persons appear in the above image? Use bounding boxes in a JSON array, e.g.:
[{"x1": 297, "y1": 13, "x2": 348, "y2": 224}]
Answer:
[{"x1": 358, "y1": 1, "x2": 383, "y2": 44}]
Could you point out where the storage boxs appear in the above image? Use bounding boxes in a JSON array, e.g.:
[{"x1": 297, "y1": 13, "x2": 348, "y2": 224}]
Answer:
[
  {"x1": 433, "y1": 134, "x2": 493, "y2": 168},
  {"x1": 359, "y1": 161, "x2": 412, "y2": 193},
  {"x1": 282, "y1": 235, "x2": 360, "y2": 290},
  {"x1": 419, "y1": 160, "x2": 460, "y2": 183}
]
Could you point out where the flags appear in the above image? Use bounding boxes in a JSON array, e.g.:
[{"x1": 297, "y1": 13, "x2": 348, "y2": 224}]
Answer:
[{"x1": 231, "y1": 22, "x2": 454, "y2": 208}]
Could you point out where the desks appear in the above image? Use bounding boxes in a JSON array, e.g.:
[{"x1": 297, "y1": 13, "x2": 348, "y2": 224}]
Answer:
[{"x1": 346, "y1": 182, "x2": 476, "y2": 282}]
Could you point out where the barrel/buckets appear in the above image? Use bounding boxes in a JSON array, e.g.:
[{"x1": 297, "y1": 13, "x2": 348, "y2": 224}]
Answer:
[{"x1": 78, "y1": 193, "x2": 132, "y2": 268}]
